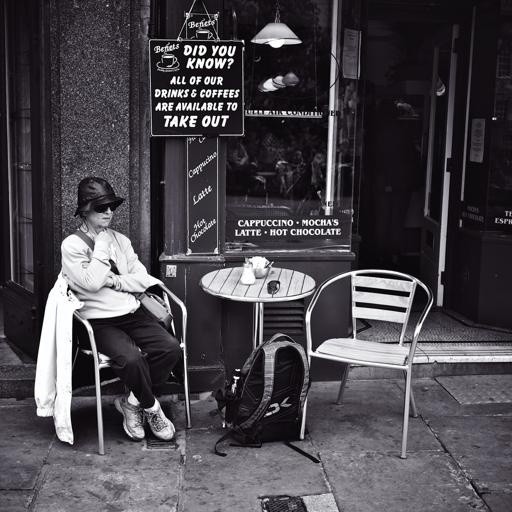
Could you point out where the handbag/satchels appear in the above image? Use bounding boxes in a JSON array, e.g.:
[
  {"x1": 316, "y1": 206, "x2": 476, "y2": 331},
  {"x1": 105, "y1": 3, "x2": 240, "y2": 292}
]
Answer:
[{"x1": 139, "y1": 291, "x2": 172, "y2": 331}]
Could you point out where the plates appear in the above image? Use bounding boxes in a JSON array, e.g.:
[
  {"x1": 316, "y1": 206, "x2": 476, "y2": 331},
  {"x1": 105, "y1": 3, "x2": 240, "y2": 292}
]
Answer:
[{"x1": 156, "y1": 62, "x2": 180, "y2": 70}]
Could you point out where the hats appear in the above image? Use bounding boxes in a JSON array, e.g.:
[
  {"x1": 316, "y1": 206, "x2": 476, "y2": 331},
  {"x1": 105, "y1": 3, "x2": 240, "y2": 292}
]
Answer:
[{"x1": 74, "y1": 177, "x2": 123, "y2": 218}]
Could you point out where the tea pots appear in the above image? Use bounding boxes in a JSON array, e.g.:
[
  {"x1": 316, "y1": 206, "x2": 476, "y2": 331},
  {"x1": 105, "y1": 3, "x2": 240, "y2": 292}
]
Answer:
[{"x1": 245, "y1": 256, "x2": 274, "y2": 278}]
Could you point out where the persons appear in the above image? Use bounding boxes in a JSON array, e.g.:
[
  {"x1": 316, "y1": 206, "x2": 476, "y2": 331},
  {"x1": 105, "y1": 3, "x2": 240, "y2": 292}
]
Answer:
[
  {"x1": 229, "y1": 126, "x2": 327, "y2": 198},
  {"x1": 61, "y1": 176, "x2": 182, "y2": 441}
]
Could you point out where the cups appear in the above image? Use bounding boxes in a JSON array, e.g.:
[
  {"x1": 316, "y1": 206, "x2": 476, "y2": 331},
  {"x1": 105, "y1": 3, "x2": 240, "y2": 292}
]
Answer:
[
  {"x1": 196, "y1": 30, "x2": 213, "y2": 39},
  {"x1": 162, "y1": 54, "x2": 177, "y2": 66}
]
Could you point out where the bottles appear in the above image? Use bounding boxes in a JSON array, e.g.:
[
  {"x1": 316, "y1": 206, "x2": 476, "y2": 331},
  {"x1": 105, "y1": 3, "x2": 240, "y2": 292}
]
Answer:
[{"x1": 240, "y1": 263, "x2": 256, "y2": 284}]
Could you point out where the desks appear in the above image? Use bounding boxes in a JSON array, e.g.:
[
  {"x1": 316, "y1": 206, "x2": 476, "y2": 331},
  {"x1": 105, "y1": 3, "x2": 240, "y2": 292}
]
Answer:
[{"x1": 199, "y1": 267, "x2": 318, "y2": 349}]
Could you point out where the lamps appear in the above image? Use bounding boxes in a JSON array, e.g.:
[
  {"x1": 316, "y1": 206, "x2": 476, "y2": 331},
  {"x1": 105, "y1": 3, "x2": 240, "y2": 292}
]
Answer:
[
  {"x1": 256, "y1": 72, "x2": 301, "y2": 93},
  {"x1": 250, "y1": 0, "x2": 302, "y2": 48}
]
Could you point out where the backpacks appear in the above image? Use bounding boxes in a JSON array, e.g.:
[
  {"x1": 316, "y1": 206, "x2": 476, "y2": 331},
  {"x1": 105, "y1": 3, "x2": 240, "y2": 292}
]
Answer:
[{"x1": 225, "y1": 332, "x2": 312, "y2": 447}]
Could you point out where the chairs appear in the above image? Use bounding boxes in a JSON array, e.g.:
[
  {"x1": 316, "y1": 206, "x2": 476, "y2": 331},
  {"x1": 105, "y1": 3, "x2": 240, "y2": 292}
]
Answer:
[
  {"x1": 299, "y1": 269, "x2": 434, "y2": 460},
  {"x1": 34, "y1": 272, "x2": 192, "y2": 456}
]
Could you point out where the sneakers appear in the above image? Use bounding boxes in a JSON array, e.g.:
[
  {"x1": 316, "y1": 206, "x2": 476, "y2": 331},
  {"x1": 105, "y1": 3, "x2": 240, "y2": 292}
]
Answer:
[
  {"x1": 114, "y1": 391, "x2": 146, "y2": 442},
  {"x1": 144, "y1": 395, "x2": 176, "y2": 442}
]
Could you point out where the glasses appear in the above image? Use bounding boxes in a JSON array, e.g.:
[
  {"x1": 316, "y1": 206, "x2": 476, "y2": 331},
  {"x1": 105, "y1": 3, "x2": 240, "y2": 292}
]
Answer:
[{"x1": 94, "y1": 202, "x2": 116, "y2": 213}]
